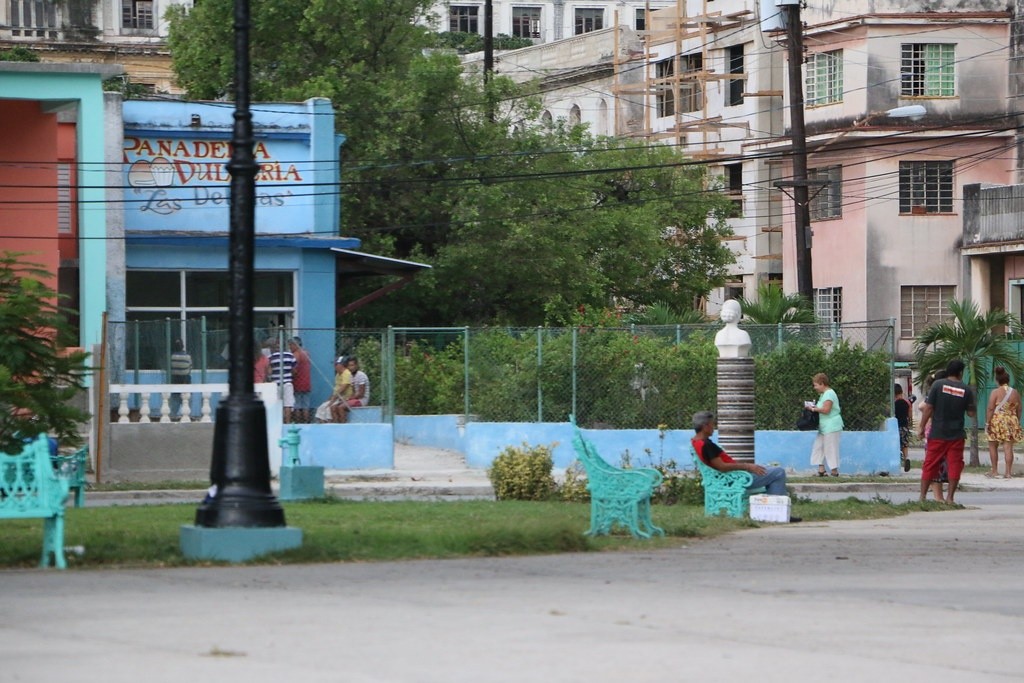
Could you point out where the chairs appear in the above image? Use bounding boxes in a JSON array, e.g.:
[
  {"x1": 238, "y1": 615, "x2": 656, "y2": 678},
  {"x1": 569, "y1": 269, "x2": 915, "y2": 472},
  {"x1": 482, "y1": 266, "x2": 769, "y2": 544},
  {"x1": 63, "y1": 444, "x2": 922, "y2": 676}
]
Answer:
[
  {"x1": 0, "y1": 433, "x2": 87, "y2": 568},
  {"x1": 569, "y1": 414, "x2": 665, "y2": 541},
  {"x1": 687, "y1": 431, "x2": 765, "y2": 518}
]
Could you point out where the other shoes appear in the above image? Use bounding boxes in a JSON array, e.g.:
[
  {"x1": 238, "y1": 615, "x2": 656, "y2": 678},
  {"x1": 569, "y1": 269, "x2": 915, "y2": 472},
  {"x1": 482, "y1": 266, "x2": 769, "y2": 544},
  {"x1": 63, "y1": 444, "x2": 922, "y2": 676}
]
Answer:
[
  {"x1": 791, "y1": 517, "x2": 802, "y2": 522},
  {"x1": 985, "y1": 474, "x2": 1000, "y2": 479},
  {"x1": 831, "y1": 474, "x2": 839, "y2": 477},
  {"x1": 1004, "y1": 477, "x2": 1013, "y2": 480},
  {"x1": 905, "y1": 459, "x2": 910, "y2": 472},
  {"x1": 813, "y1": 471, "x2": 828, "y2": 476}
]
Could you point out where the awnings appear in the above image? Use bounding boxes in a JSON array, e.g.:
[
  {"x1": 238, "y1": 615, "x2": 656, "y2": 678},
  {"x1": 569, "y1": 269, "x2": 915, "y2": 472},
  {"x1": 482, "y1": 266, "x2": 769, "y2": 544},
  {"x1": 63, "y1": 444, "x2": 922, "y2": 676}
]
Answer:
[{"x1": 331, "y1": 247, "x2": 432, "y2": 316}]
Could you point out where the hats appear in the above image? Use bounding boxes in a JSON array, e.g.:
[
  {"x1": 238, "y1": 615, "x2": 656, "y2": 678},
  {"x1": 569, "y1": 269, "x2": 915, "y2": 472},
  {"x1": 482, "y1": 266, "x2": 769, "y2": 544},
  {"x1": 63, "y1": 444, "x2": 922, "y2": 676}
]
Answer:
[{"x1": 335, "y1": 356, "x2": 344, "y2": 364}]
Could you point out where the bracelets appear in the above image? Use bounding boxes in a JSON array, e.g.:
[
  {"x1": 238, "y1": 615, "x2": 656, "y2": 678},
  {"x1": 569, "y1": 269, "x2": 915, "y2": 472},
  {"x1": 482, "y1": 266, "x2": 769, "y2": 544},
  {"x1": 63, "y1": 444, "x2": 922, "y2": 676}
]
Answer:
[
  {"x1": 812, "y1": 408, "x2": 814, "y2": 412},
  {"x1": 985, "y1": 423, "x2": 989, "y2": 426}
]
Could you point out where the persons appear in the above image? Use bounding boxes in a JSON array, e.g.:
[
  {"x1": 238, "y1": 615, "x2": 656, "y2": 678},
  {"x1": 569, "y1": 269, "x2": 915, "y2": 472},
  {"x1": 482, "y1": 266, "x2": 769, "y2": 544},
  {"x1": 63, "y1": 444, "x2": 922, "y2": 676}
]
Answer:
[
  {"x1": 917, "y1": 359, "x2": 976, "y2": 503},
  {"x1": 253, "y1": 337, "x2": 295, "y2": 424},
  {"x1": 714, "y1": 300, "x2": 752, "y2": 358},
  {"x1": 315, "y1": 356, "x2": 354, "y2": 423},
  {"x1": 984, "y1": 366, "x2": 1024, "y2": 478},
  {"x1": 894, "y1": 383, "x2": 912, "y2": 472},
  {"x1": 806, "y1": 373, "x2": 845, "y2": 477},
  {"x1": 691, "y1": 411, "x2": 786, "y2": 495},
  {"x1": 287, "y1": 336, "x2": 312, "y2": 424},
  {"x1": 331, "y1": 356, "x2": 369, "y2": 423},
  {"x1": 170, "y1": 338, "x2": 192, "y2": 416}
]
